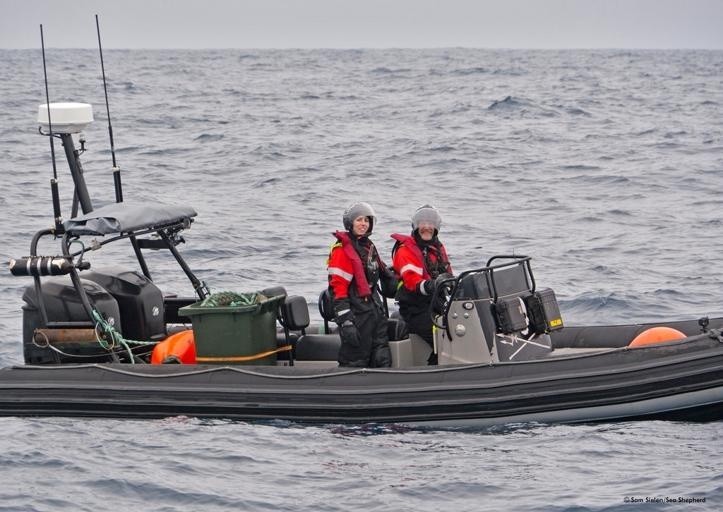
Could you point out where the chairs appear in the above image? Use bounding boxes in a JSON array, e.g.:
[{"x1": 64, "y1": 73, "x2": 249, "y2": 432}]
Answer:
[{"x1": 262, "y1": 286, "x2": 340, "y2": 367}]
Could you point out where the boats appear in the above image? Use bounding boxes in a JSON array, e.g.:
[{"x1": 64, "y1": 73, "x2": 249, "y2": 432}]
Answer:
[{"x1": 1, "y1": 265, "x2": 722, "y2": 431}]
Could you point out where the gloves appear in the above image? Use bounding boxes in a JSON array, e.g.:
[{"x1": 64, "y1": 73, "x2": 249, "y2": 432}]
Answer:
[
  {"x1": 333, "y1": 310, "x2": 362, "y2": 348},
  {"x1": 425, "y1": 273, "x2": 453, "y2": 296}
]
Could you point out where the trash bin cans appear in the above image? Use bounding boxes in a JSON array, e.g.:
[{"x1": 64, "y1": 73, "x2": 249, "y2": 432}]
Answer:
[{"x1": 178, "y1": 292, "x2": 285, "y2": 365}]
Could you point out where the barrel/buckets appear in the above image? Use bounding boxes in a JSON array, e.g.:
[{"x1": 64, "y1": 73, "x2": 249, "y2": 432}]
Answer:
[{"x1": 148, "y1": 329, "x2": 197, "y2": 366}]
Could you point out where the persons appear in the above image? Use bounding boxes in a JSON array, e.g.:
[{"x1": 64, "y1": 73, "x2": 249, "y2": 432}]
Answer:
[
  {"x1": 328, "y1": 201, "x2": 401, "y2": 368},
  {"x1": 390, "y1": 204, "x2": 453, "y2": 365}
]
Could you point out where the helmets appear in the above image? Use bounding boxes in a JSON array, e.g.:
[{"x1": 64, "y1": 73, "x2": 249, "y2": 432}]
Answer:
[
  {"x1": 343, "y1": 201, "x2": 377, "y2": 236},
  {"x1": 411, "y1": 203, "x2": 442, "y2": 234}
]
